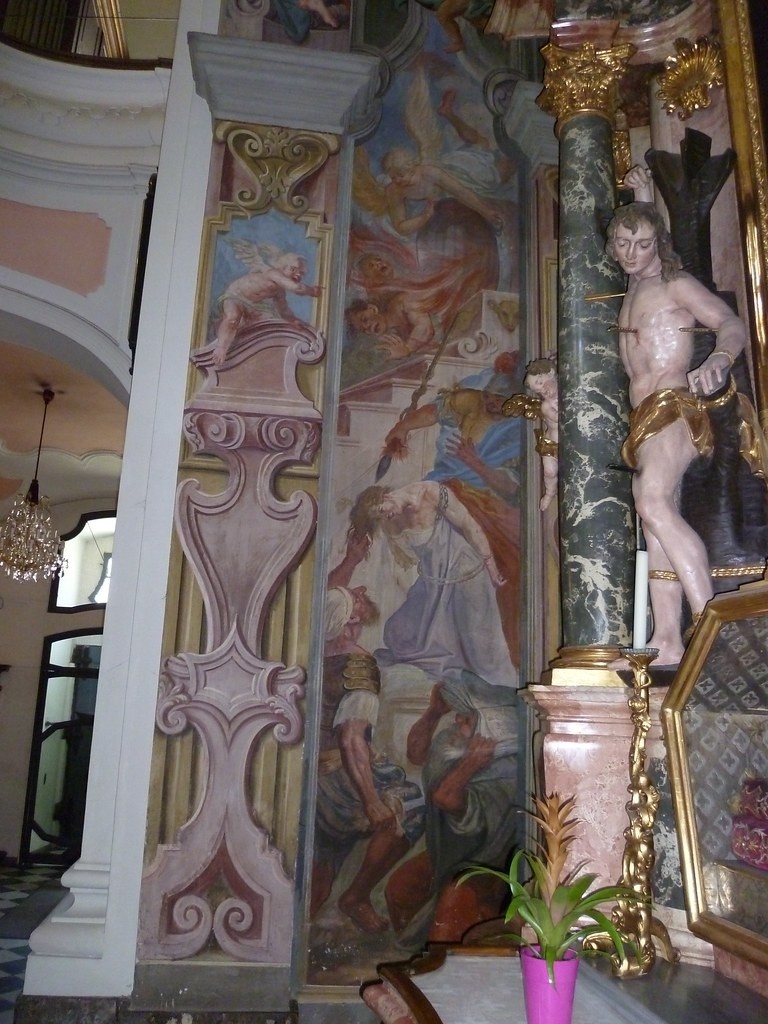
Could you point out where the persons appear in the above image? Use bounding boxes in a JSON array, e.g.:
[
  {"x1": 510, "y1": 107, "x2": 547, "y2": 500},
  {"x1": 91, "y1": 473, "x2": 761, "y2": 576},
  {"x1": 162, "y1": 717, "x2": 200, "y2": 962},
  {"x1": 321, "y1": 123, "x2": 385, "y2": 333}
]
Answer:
[{"x1": 606, "y1": 164, "x2": 746, "y2": 674}]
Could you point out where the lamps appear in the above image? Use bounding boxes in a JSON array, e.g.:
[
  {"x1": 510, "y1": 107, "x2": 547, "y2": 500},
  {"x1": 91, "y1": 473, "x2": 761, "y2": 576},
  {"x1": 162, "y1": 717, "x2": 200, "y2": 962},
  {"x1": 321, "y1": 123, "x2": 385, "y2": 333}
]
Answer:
[{"x1": 0, "y1": 389, "x2": 70, "y2": 584}]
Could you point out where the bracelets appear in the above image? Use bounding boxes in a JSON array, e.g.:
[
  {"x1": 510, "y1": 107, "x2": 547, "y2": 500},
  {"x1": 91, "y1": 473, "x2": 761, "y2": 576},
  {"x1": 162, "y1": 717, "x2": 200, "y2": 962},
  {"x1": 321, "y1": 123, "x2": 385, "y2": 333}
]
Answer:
[{"x1": 708, "y1": 350, "x2": 734, "y2": 366}]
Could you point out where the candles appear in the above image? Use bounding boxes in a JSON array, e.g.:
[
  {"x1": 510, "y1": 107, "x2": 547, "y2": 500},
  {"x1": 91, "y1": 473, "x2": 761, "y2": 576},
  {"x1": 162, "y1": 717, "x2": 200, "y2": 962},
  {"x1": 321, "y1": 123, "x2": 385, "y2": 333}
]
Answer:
[{"x1": 632, "y1": 549, "x2": 649, "y2": 647}]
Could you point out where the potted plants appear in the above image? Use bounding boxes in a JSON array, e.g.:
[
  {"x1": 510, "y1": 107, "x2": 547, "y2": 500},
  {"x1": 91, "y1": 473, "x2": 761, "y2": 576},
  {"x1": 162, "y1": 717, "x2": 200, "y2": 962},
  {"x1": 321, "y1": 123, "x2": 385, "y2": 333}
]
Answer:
[{"x1": 457, "y1": 790, "x2": 656, "y2": 1024}]
